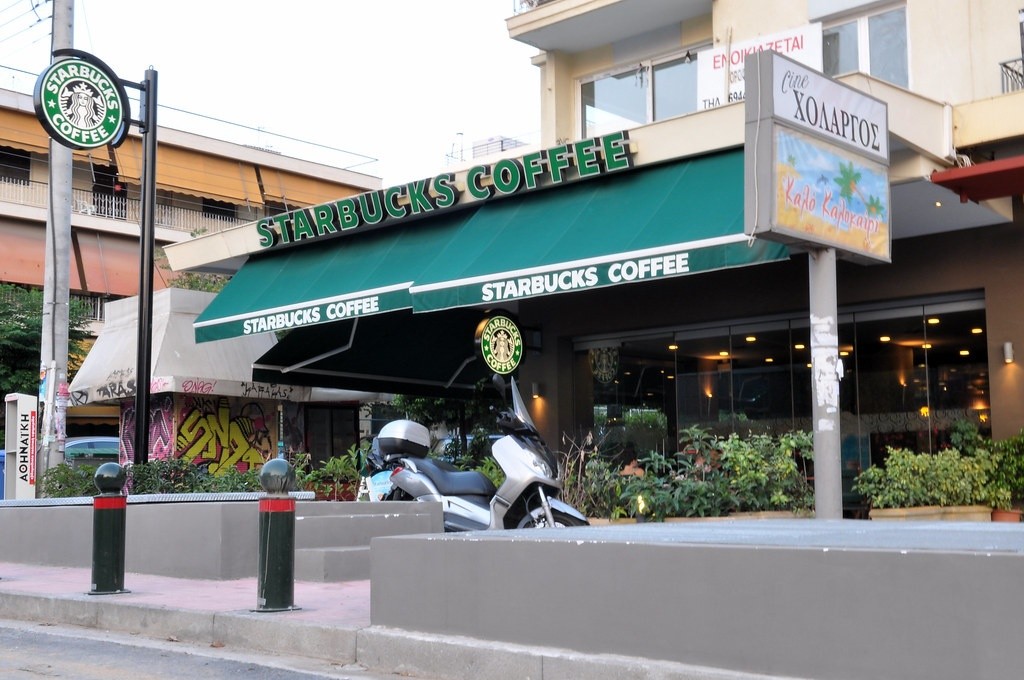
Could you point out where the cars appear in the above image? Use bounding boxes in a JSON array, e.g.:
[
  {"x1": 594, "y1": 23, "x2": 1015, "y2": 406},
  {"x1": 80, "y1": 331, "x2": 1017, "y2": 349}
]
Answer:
[
  {"x1": 430, "y1": 432, "x2": 507, "y2": 462},
  {"x1": 64, "y1": 436, "x2": 121, "y2": 449}
]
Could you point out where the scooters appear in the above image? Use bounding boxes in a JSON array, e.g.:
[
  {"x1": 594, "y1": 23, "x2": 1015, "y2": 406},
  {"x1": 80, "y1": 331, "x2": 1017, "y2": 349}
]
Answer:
[{"x1": 355, "y1": 374, "x2": 590, "y2": 535}]
